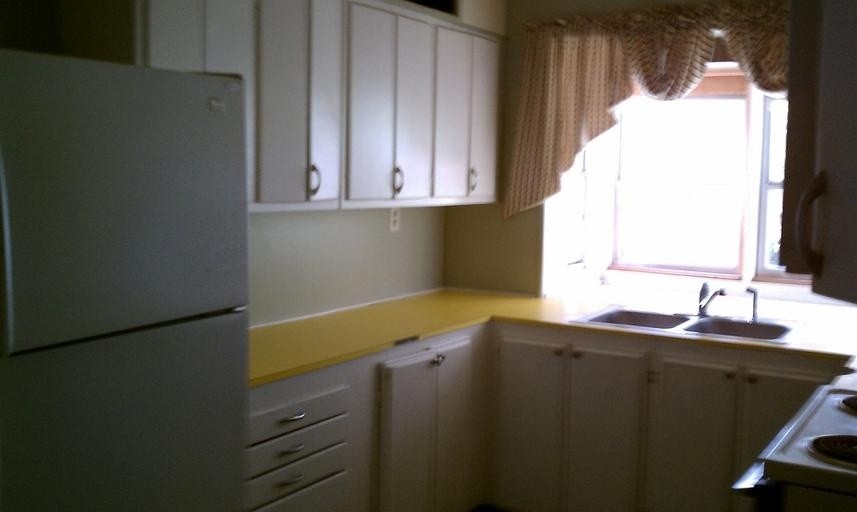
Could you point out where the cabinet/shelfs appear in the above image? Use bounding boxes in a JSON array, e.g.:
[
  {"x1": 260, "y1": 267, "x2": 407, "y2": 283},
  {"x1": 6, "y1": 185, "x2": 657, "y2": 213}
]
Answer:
[
  {"x1": 60, "y1": 1, "x2": 255, "y2": 209},
  {"x1": 242, "y1": 364, "x2": 378, "y2": 511},
  {"x1": 256, "y1": 0, "x2": 350, "y2": 210},
  {"x1": 649, "y1": 334, "x2": 827, "y2": 511},
  {"x1": 350, "y1": 3, "x2": 434, "y2": 207},
  {"x1": 434, "y1": 24, "x2": 496, "y2": 207},
  {"x1": 377, "y1": 321, "x2": 495, "y2": 512},
  {"x1": 495, "y1": 316, "x2": 651, "y2": 512}
]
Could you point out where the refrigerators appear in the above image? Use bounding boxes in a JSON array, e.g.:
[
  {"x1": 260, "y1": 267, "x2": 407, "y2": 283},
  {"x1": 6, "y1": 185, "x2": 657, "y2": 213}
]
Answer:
[{"x1": 1, "y1": 52, "x2": 250, "y2": 511}]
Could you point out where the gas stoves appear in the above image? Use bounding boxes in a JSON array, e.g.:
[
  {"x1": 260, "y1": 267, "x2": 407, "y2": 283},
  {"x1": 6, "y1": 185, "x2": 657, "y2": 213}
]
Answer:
[{"x1": 756, "y1": 384, "x2": 857, "y2": 491}]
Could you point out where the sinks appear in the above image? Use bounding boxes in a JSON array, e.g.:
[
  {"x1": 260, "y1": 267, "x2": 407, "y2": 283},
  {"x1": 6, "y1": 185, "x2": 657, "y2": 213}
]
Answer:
[
  {"x1": 588, "y1": 305, "x2": 691, "y2": 332},
  {"x1": 682, "y1": 313, "x2": 792, "y2": 343}
]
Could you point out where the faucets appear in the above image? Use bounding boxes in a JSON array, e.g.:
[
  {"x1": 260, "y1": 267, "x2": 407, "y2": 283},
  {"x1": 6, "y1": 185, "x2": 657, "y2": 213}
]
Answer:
[
  {"x1": 746, "y1": 283, "x2": 761, "y2": 323},
  {"x1": 698, "y1": 282, "x2": 728, "y2": 314}
]
